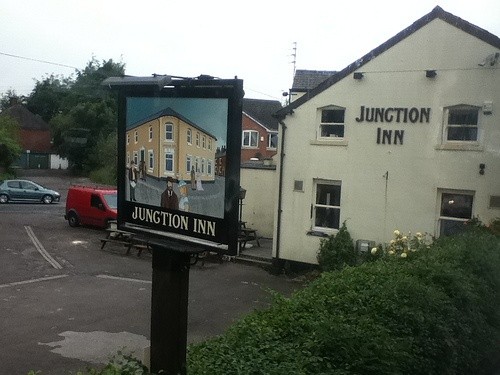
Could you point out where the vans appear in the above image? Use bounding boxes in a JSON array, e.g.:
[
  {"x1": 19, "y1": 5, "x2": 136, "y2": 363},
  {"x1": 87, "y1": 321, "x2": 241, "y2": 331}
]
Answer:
[{"x1": 63, "y1": 182, "x2": 117, "y2": 229}]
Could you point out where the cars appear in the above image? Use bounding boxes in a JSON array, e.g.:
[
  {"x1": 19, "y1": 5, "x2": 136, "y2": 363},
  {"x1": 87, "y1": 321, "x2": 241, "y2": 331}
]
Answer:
[{"x1": 0, "y1": 179, "x2": 62, "y2": 205}]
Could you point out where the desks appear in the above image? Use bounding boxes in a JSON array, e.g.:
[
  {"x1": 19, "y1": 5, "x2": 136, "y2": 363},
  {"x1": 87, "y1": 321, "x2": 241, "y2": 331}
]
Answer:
[
  {"x1": 106, "y1": 228, "x2": 138, "y2": 244},
  {"x1": 242, "y1": 229, "x2": 260, "y2": 250}
]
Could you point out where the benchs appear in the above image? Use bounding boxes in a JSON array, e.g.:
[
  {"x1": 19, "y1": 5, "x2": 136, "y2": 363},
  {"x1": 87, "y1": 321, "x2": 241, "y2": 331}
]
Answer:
[{"x1": 100, "y1": 227, "x2": 261, "y2": 265}]
